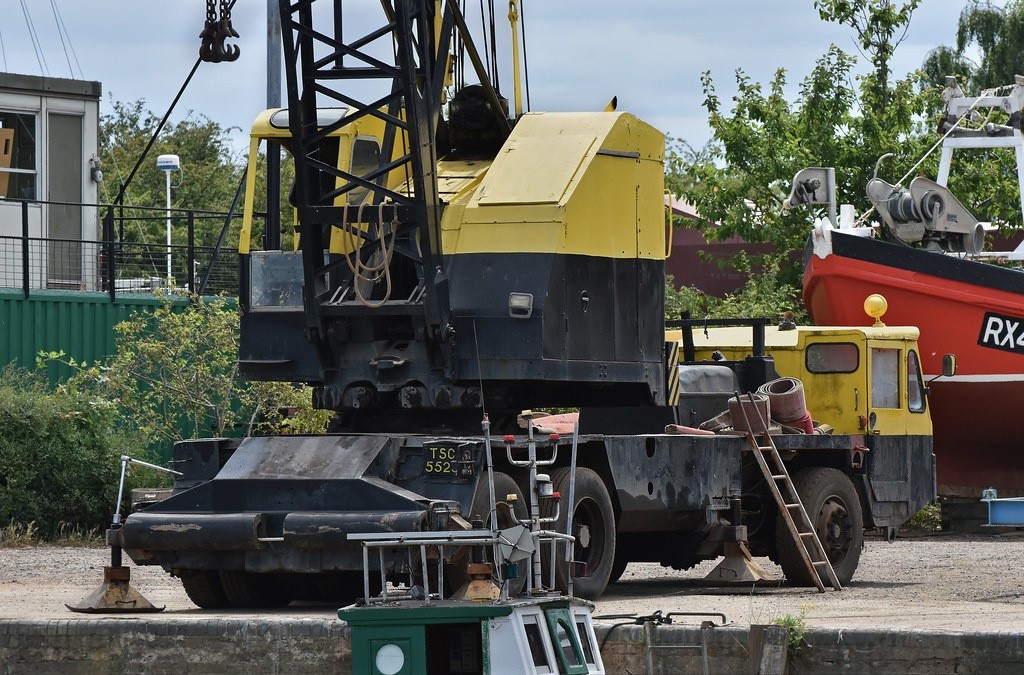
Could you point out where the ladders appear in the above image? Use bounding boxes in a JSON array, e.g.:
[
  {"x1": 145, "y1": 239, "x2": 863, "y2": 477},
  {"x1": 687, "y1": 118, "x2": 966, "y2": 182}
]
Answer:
[{"x1": 729, "y1": 391, "x2": 846, "y2": 593}]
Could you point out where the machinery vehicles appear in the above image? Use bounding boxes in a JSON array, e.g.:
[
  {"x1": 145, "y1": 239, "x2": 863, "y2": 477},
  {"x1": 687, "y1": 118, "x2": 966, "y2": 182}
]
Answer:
[{"x1": 66, "y1": 0, "x2": 958, "y2": 622}]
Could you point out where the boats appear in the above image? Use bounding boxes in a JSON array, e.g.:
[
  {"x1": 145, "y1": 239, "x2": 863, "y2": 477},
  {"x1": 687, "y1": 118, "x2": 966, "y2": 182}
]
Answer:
[{"x1": 779, "y1": 72, "x2": 1024, "y2": 504}]
